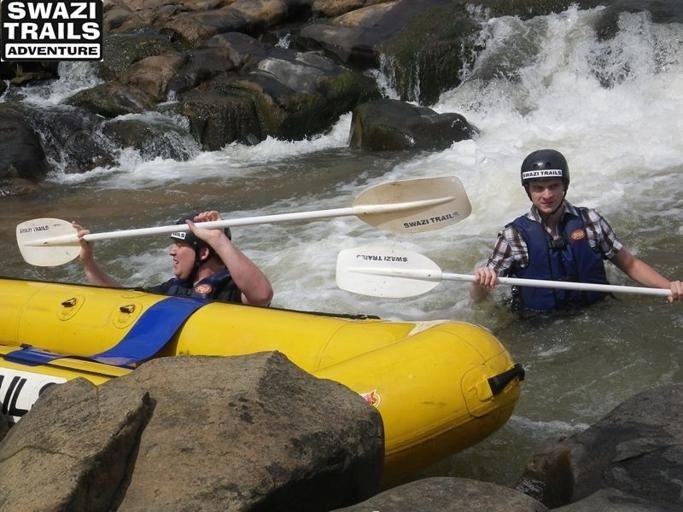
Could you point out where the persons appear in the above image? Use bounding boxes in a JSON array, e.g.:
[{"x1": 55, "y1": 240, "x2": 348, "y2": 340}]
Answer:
[
  {"x1": 68, "y1": 209, "x2": 272, "y2": 307},
  {"x1": 467, "y1": 147, "x2": 682, "y2": 324}
]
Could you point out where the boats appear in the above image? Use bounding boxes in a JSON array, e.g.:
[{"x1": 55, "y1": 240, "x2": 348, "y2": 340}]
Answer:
[{"x1": 0, "y1": 279, "x2": 523, "y2": 485}]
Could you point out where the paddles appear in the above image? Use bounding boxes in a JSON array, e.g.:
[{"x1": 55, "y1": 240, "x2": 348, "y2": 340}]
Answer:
[
  {"x1": 336, "y1": 244, "x2": 674, "y2": 300},
  {"x1": 14, "y1": 175, "x2": 471, "y2": 269}
]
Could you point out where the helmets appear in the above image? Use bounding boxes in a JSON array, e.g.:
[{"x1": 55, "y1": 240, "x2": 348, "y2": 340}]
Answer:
[
  {"x1": 171, "y1": 214, "x2": 231, "y2": 249},
  {"x1": 521, "y1": 150, "x2": 569, "y2": 188}
]
